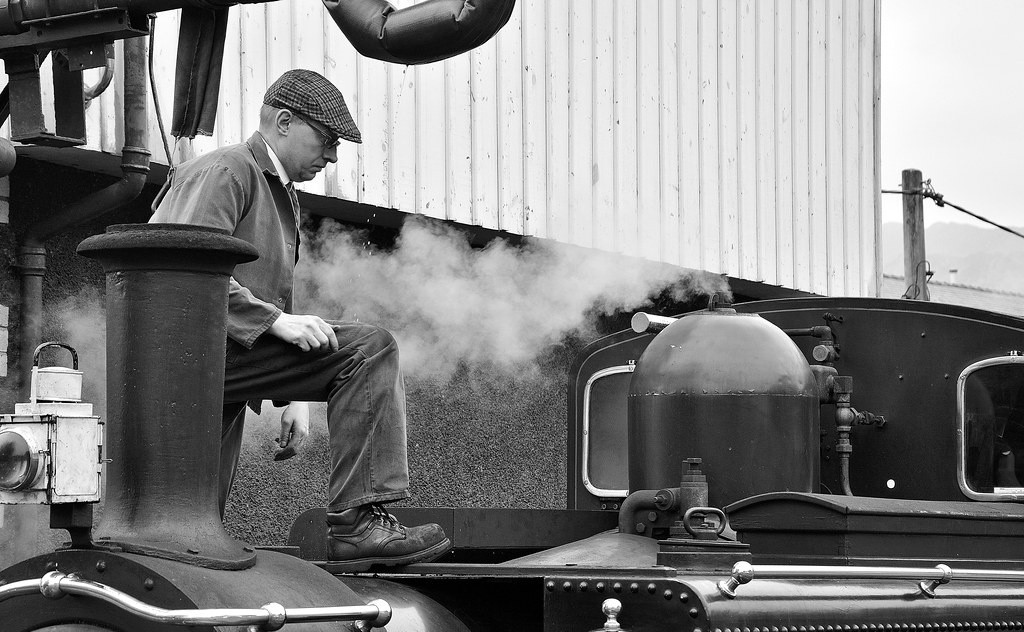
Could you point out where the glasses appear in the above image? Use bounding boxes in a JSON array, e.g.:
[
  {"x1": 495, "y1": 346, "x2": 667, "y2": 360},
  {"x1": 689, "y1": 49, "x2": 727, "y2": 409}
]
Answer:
[{"x1": 292, "y1": 111, "x2": 341, "y2": 149}]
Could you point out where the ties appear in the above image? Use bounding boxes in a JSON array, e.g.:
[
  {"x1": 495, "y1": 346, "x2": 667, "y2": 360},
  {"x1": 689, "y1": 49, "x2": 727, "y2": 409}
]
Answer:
[{"x1": 286, "y1": 183, "x2": 301, "y2": 265}]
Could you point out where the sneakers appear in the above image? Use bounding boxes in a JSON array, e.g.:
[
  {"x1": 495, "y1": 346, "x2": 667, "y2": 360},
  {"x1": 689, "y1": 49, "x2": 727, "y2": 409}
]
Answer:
[{"x1": 325, "y1": 502, "x2": 452, "y2": 574}]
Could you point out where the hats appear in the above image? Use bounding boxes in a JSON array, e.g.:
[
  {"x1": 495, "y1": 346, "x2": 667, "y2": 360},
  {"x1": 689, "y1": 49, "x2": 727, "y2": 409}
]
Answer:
[{"x1": 263, "y1": 69, "x2": 362, "y2": 144}]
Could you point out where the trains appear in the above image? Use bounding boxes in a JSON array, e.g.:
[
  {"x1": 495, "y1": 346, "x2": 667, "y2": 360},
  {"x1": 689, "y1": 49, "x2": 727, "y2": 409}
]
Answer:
[{"x1": 0, "y1": 223, "x2": 1023, "y2": 632}]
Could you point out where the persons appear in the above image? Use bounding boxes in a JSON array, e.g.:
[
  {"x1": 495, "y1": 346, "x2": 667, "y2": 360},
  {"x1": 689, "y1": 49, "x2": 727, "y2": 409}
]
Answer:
[{"x1": 145, "y1": 67, "x2": 452, "y2": 574}]
